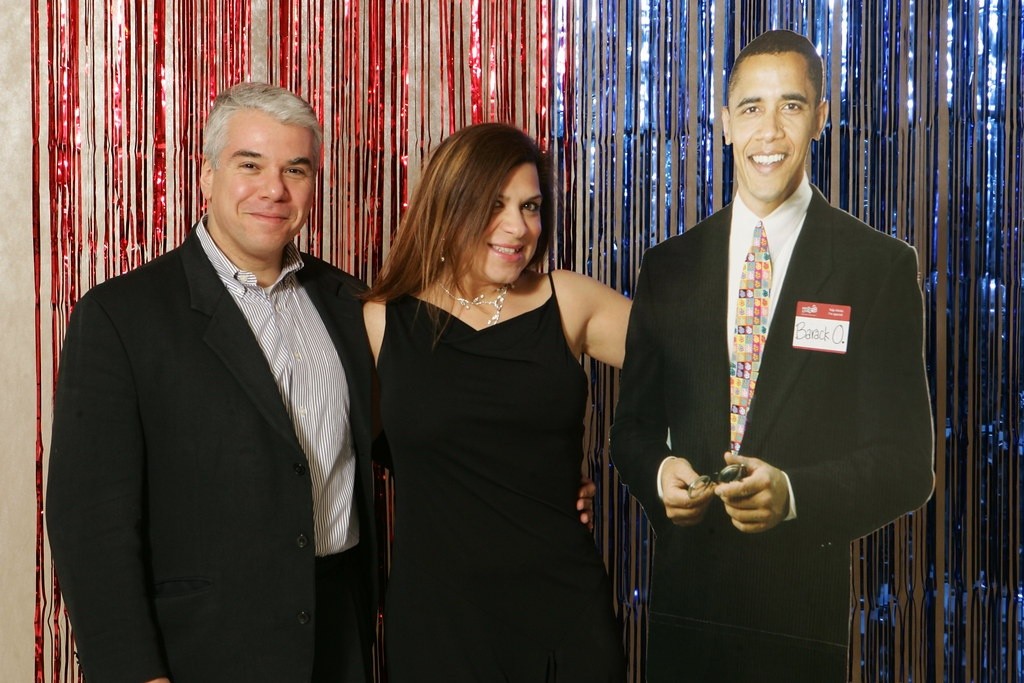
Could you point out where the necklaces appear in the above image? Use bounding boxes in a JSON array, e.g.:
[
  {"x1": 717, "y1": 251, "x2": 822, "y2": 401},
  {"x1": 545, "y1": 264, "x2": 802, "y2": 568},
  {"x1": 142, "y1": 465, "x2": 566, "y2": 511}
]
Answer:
[{"x1": 434, "y1": 279, "x2": 515, "y2": 325}]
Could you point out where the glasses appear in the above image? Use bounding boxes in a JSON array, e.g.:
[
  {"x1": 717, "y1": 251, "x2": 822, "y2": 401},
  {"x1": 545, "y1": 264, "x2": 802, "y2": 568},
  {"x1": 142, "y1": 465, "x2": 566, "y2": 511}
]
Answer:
[{"x1": 686, "y1": 463, "x2": 748, "y2": 499}]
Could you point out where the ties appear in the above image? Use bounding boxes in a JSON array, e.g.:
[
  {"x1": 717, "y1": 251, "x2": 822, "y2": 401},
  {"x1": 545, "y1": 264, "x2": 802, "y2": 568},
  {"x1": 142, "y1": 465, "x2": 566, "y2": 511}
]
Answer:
[{"x1": 729, "y1": 221, "x2": 773, "y2": 456}]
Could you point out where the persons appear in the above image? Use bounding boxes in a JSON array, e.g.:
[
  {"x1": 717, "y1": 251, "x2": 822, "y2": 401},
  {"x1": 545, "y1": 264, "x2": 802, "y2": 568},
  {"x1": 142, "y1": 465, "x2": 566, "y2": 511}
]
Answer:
[
  {"x1": 608, "y1": 30, "x2": 936, "y2": 683},
  {"x1": 46, "y1": 82, "x2": 633, "y2": 683}
]
